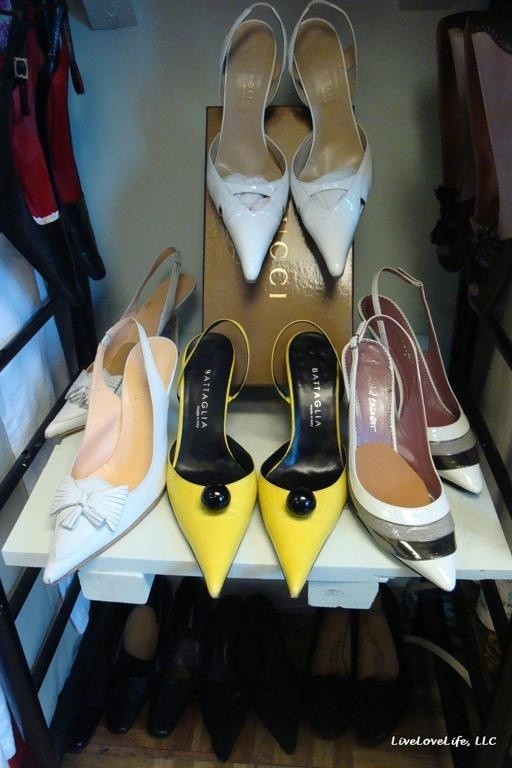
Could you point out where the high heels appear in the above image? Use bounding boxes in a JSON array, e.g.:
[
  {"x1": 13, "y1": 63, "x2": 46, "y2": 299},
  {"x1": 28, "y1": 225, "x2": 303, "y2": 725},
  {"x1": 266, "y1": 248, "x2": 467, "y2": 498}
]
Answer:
[
  {"x1": 467, "y1": 29, "x2": 512, "y2": 310},
  {"x1": 432, "y1": 13, "x2": 477, "y2": 271},
  {"x1": 339, "y1": 314, "x2": 460, "y2": 594},
  {"x1": 287, "y1": 4, "x2": 375, "y2": 281},
  {"x1": 162, "y1": 316, "x2": 261, "y2": 601},
  {"x1": 348, "y1": 579, "x2": 410, "y2": 745},
  {"x1": 255, "y1": 317, "x2": 350, "y2": 600},
  {"x1": 237, "y1": 601, "x2": 307, "y2": 756},
  {"x1": 304, "y1": 601, "x2": 361, "y2": 744},
  {"x1": 29, "y1": 1, "x2": 106, "y2": 290},
  {"x1": 360, "y1": 265, "x2": 487, "y2": 499},
  {"x1": 206, "y1": 0, "x2": 290, "y2": 283},
  {"x1": 3, "y1": 18, "x2": 91, "y2": 300},
  {"x1": 35, "y1": 243, "x2": 197, "y2": 446},
  {"x1": 143, "y1": 572, "x2": 202, "y2": 745},
  {"x1": 32, "y1": 316, "x2": 168, "y2": 595},
  {"x1": 101, "y1": 600, "x2": 163, "y2": 739},
  {"x1": 199, "y1": 593, "x2": 248, "y2": 765}
]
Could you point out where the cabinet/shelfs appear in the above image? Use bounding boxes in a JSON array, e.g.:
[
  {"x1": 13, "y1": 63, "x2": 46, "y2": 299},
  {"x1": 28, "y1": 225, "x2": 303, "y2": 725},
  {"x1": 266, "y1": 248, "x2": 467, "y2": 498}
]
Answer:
[
  {"x1": 443, "y1": 217, "x2": 512, "y2": 763},
  {"x1": 0, "y1": 164, "x2": 104, "y2": 768}
]
